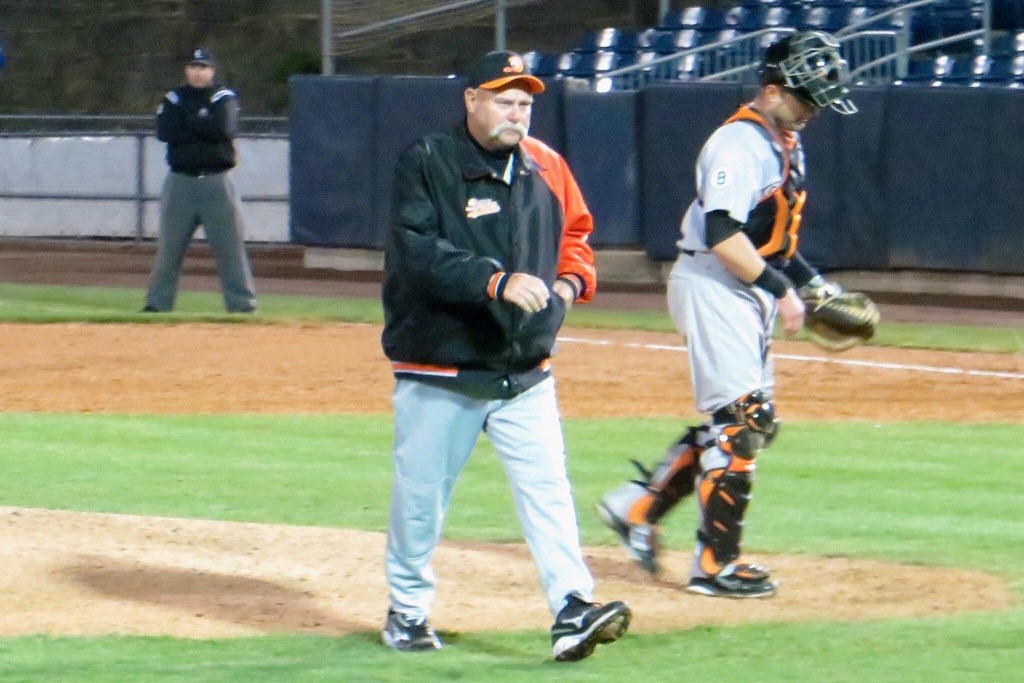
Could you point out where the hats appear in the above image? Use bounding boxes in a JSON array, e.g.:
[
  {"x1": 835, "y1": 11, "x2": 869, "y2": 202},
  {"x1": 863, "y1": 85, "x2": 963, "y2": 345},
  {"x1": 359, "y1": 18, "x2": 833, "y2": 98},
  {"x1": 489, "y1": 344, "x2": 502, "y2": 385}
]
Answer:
[
  {"x1": 183, "y1": 46, "x2": 215, "y2": 66},
  {"x1": 758, "y1": 32, "x2": 861, "y2": 114},
  {"x1": 466, "y1": 51, "x2": 544, "y2": 95}
]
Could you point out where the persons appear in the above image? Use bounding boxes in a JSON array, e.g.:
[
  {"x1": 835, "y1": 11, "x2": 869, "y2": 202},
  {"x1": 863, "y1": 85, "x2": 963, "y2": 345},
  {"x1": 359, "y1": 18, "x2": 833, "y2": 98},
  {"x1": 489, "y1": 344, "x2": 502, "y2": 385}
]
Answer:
[
  {"x1": 140, "y1": 46, "x2": 258, "y2": 313},
  {"x1": 380, "y1": 48, "x2": 634, "y2": 663},
  {"x1": 592, "y1": 28, "x2": 882, "y2": 598}
]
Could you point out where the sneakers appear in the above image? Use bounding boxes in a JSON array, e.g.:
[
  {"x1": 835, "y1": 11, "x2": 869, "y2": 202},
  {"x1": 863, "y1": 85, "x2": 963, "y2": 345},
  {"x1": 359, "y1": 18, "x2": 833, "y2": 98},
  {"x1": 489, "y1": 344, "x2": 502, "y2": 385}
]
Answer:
[
  {"x1": 686, "y1": 561, "x2": 778, "y2": 598},
  {"x1": 550, "y1": 598, "x2": 633, "y2": 663},
  {"x1": 382, "y1": 607, "x2": 442, "y2": 651},
  {"x1": 596, "y1": 495, "x2": 658, "y2": 572}
]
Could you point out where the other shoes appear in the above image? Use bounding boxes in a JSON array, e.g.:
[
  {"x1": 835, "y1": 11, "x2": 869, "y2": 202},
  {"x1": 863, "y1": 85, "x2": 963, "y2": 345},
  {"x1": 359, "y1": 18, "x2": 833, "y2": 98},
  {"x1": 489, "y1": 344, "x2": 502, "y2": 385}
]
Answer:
[{"x1": 141, "y1": 306, "x2": 158, "y2": 313}]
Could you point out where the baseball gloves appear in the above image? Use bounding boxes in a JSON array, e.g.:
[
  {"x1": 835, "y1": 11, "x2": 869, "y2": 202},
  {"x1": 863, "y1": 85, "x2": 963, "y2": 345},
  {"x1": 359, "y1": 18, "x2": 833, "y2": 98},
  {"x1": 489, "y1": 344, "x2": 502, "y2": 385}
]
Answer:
[{"x1": 802, "y1": 281, "x2": 881, "y2": 350}]
